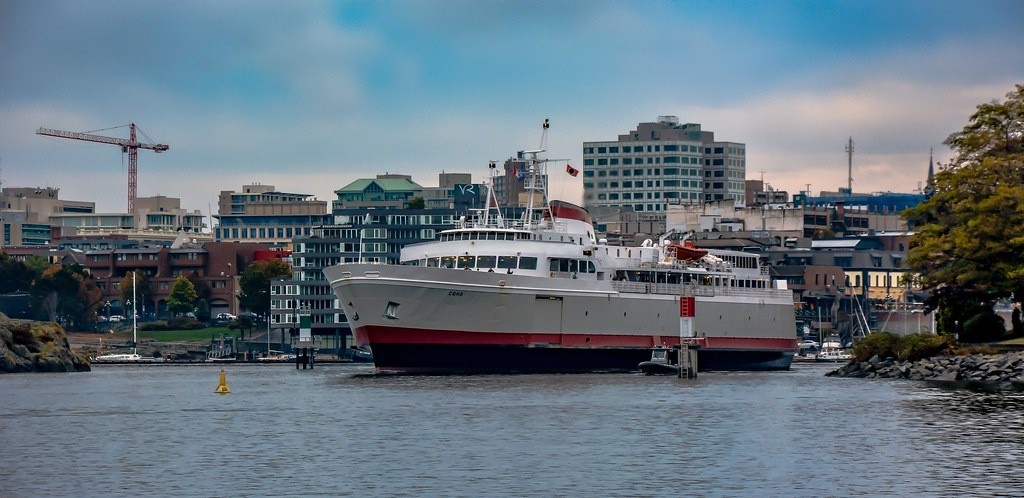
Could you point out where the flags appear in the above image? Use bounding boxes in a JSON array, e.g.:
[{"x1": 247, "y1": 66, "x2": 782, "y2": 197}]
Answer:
[
  {"x1": 513, "y1": 166, "x2": 522, "y2": 180},
  {"x1": 567, "y1": 164, "x2": 579, "y2": 177}
]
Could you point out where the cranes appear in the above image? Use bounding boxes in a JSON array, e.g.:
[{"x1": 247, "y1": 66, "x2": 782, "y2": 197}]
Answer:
[{"x1": 34, "y1": 122, "x2": 170, "y2": 214}]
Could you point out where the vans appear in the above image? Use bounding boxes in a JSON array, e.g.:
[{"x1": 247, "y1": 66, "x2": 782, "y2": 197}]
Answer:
[{"x1": 823, "y1": 336, "x2": 841, "y2": 350}]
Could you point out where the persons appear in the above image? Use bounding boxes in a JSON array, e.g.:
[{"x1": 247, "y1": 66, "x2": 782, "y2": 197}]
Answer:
[
  {"x1": 441, "y1": 263, "x2": 513, "y2": 275},
  {"x1": 573, "y1": 271, "x2": 578, "y2": 279},
  {"x1": 612, "y1": 276, "x2": 627, "y2": 281}
]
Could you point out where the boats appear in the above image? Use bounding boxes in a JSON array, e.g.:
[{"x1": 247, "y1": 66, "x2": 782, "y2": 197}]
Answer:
[
  {"x1": 351, "y1": 349, "x2": 373, "y2": 364},
  {"x1": 638, "y1": 344, "x2": 679, "y2": 374},
  {"x1": 816, "y1": 351, "x2": 853, "y2": 363},
  {"x1": 322, "y1": 119, "x2": 799, "y2": 381}
]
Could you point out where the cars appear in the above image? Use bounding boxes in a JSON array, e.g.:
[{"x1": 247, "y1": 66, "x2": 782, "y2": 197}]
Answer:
[
  {"x1": 797, "y1": 339, "x2": 819, "y2": 348},
  {"x1": 97, "y1": 315, "x2": 126, "y2": 323},
  {"x1": 217, "y1": 313, "x2": 238, "y2": 320}
]
